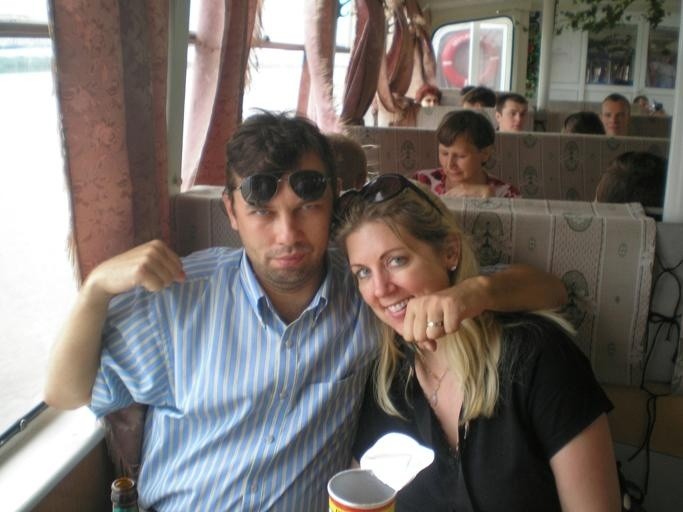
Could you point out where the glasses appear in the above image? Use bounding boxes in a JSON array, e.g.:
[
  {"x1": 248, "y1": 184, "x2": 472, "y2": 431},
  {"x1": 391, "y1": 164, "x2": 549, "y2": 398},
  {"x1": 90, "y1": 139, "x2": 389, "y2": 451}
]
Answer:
[
  {"x1": 336, "y1": 173, "x2": 442, "y2": 216},
  {"x1": 235, "y1": 170, "x2": 332, "y2": 207}
]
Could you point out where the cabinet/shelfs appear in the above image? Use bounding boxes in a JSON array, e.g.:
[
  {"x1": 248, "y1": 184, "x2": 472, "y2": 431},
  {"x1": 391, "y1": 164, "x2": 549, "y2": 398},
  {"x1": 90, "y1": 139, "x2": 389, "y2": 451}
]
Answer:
[{"x1": 580, "y1": 14, "x2": 681, "y2": 93}]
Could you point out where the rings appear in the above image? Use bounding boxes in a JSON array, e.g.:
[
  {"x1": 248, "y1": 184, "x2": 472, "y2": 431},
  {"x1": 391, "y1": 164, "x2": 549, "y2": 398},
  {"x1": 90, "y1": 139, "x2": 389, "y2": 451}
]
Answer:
[{"x1": 425, "y1": 321, "x2": 445, "y2": 328}]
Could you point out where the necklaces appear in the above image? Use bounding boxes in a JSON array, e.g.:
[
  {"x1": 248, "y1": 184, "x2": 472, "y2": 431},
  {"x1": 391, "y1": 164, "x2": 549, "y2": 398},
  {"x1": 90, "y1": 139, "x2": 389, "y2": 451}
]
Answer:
[{"x1": 419, "y1": 362, "x2": 451, "y2": 407}]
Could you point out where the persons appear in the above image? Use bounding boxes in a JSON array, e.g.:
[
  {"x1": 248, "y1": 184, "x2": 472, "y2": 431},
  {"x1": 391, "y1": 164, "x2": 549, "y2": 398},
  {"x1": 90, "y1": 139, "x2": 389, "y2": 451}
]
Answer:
[
  {"x1": 39, "y1": 114, "x2": 568, "y2": 512},
  {"x1": 415, "y1": 84, "x2": 442, "y2": 107},
  {"x1": 561, "y1": 111, "x2": 606, "y2": 136},
  {"x1": 405, "y1": 109, "x2": 522, "y2": 200},
  {"x1": 460, "y1": 86, "x2": 495, "y2": 110},
  {"x1": 600, "y1": 93, "x2": 632, "y2": 135},
  {"x1": 494, "y1": 93, "x2": 528, "y2": 131},
  {"x1": 633, "y1": 95, "x2": 650, "y2": 110},
  {"x1": 320, "y1": 130, "x2": 369, "y2": 197},
  {"x1": 593, "y1": 151, "x2": 667, "y2": 221},
  {"x1": 328, "y1": 175, "x2": 624, "y2": 512}
]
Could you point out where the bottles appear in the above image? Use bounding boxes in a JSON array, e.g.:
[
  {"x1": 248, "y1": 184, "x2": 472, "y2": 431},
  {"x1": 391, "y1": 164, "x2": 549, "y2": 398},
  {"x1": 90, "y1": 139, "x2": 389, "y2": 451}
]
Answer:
[{"x1": 110, "y1": 477, "x2": 139, "y2": 512}]
